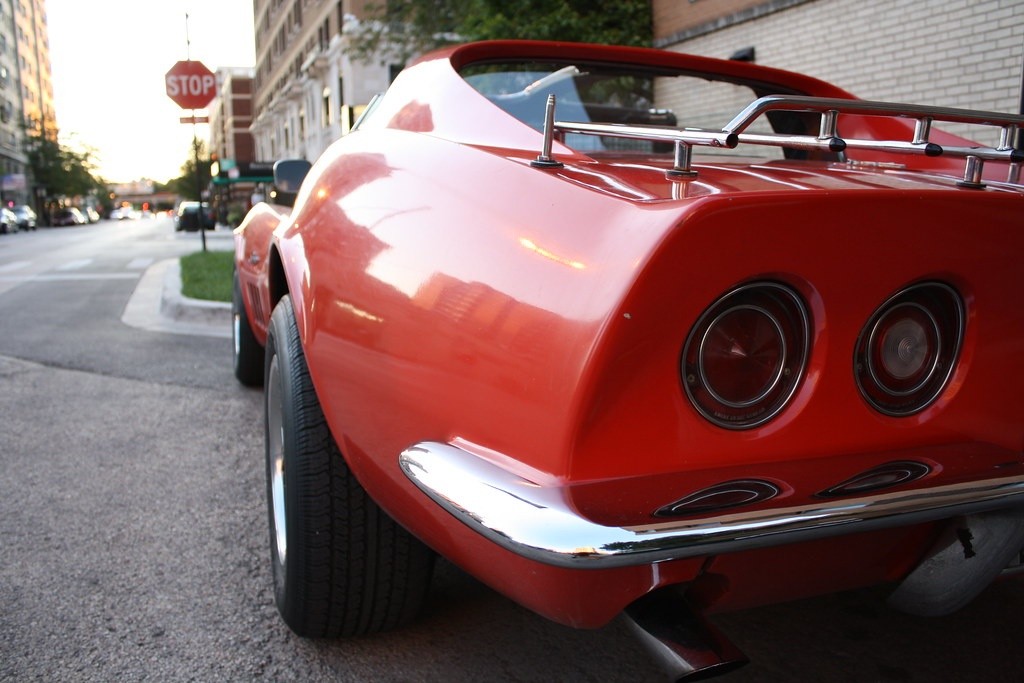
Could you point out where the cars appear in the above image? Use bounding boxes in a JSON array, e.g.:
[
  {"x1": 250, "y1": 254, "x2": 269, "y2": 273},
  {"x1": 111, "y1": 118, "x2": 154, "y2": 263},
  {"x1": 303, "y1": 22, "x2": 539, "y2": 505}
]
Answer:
[{"x1": 175, "y1": 201, "x2": 216, "y2": 231}]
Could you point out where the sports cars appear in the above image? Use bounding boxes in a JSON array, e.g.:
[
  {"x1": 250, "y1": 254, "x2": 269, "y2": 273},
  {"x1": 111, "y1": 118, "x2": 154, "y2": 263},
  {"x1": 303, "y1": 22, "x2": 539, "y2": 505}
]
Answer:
[{"x1": 234, "y1": 40, "x2": 1024, "y2": 683}]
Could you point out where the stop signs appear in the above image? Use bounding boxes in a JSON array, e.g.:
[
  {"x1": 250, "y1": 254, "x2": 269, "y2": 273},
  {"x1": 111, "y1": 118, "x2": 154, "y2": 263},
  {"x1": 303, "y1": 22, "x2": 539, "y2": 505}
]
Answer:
[{"x1": 166, "y1": 61, "x2": 217, "y2": 110}]
